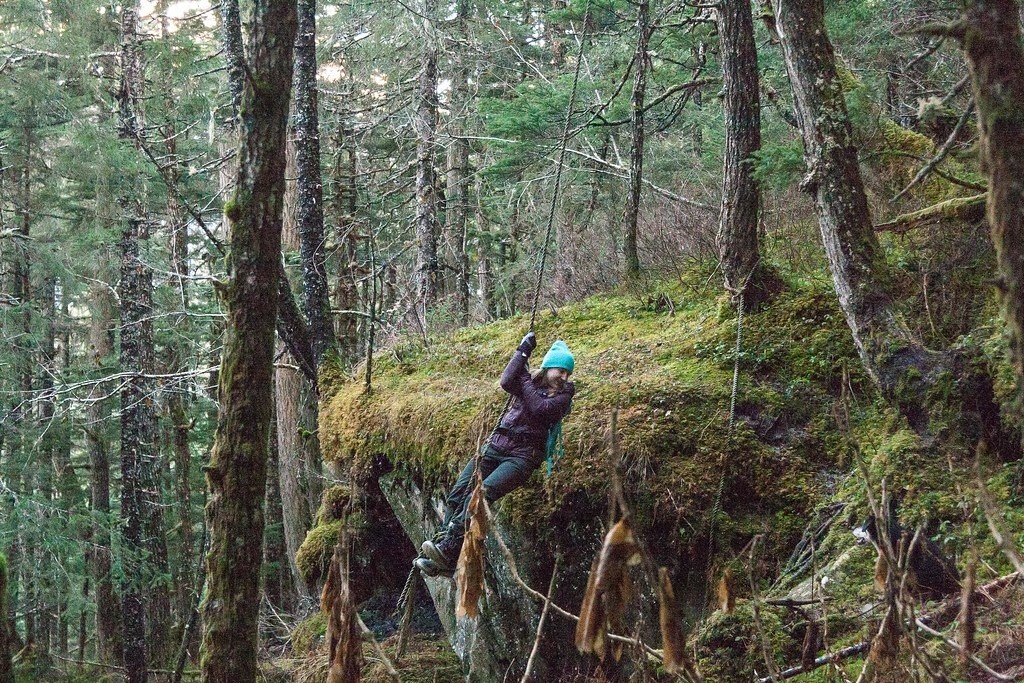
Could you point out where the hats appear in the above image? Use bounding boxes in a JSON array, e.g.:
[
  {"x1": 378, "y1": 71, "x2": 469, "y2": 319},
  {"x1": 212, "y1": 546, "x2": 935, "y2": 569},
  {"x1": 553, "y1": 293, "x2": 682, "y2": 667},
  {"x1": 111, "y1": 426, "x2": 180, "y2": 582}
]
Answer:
[{"x1": 541, "y1": 340, "x2": 574, "y2": 375}]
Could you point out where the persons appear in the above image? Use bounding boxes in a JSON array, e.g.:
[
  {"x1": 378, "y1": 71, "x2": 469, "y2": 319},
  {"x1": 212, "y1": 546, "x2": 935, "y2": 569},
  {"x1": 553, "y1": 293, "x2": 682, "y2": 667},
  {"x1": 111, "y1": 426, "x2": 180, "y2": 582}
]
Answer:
[{"x1": 415, "y1": 332, "x2": 575, "y2": 577}]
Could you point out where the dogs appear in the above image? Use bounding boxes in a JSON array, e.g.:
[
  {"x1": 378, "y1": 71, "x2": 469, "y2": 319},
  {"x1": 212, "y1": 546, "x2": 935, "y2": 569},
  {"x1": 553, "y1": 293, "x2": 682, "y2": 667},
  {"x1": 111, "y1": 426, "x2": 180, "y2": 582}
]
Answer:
[{"x1": 853, "y1": 516, "x2": 960, "y2": 594}]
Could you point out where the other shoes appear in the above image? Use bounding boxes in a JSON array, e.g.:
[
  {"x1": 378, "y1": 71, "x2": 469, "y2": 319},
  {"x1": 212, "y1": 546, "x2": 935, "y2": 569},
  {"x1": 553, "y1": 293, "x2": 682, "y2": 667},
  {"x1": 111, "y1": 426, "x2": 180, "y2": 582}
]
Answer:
[
  {"x1": 417, "y1": 557, "x2": 451, "y2": 579},
  {"x1": 421, "y1": 535, "x2": 459, "y2": 570}
]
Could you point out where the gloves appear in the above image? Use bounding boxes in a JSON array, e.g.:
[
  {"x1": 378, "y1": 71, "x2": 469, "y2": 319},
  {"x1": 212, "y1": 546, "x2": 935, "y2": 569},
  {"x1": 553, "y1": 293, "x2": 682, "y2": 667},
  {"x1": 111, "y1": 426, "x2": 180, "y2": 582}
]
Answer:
[
  {"x1": 516, "y1": 332, "x2": 536, "y2": 360},
  {"x1": 520, "y1": 363, "x2": 529, "y2": 373}
]
